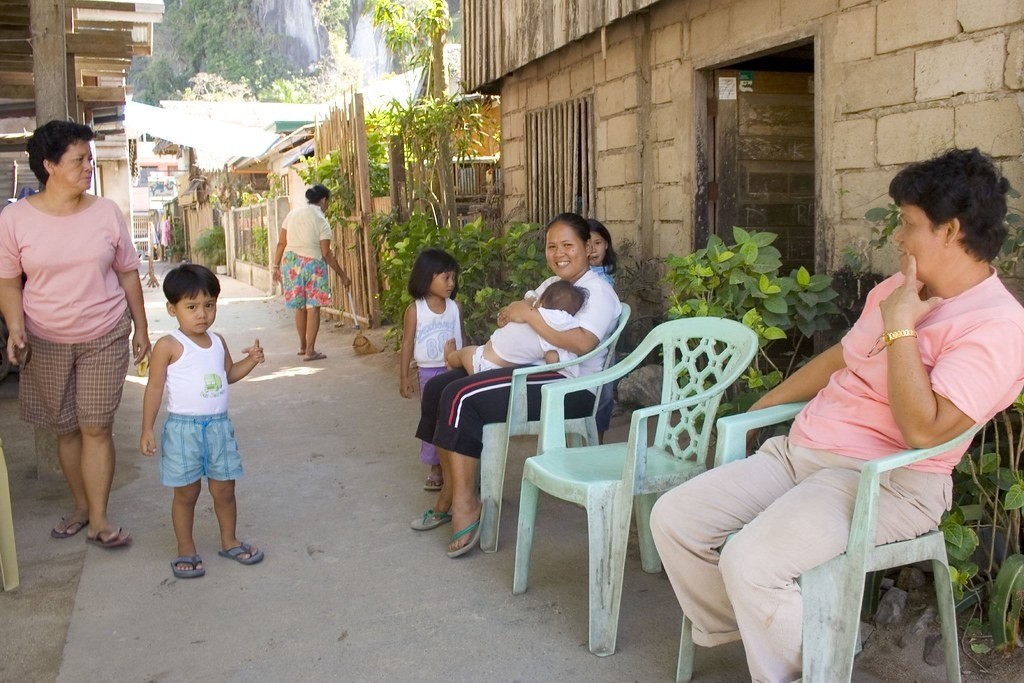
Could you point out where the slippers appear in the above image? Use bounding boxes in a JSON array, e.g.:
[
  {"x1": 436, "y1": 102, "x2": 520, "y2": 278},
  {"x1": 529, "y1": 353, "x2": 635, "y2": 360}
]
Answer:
[
  {"x1": 86, "y1": 526, "x2": 130, "y2": 547},
  {"x1": 303, "y1": 353, "x2": 326, "y2": 360},
  {"x1": 446, "y1": 502, "x2": 485, "y2": 558},
  {"x1": 51, "y1": 512, "x2": 89, "y2": 538},
  {"x1": 298, "y1": 350, "x2": 317, "y2": 355},
  {"x1": 219, "y1": 543, "x2": 264, "y2": 564},
  {"x1": 411, "y1": 509, "x2": 451, "y2": 530},
  {"x1": 171, "y1": 555, "x2": 205, "y2": 578}
]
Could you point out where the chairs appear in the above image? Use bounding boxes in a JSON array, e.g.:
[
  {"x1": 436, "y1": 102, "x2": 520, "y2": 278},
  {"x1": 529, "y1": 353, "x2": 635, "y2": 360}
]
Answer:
[
  {"x1": 676, "y1": 400, "x2": 984, "y2": 683},
  {"x1": 478, "y1": 303, "x2": 631, "y2": 554},
  {"x1": 511, "y1": 315, "x2": 760, "y2": 657}
]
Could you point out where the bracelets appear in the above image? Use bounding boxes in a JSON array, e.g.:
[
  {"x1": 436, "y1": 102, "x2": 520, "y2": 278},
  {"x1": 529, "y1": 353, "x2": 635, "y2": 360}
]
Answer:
[
  {"x1": 883, "y1": 330, "x2": 917, "y2": 344},
  {"x1": 273, "y1": 265, "x2": 280, "y2": 268}
]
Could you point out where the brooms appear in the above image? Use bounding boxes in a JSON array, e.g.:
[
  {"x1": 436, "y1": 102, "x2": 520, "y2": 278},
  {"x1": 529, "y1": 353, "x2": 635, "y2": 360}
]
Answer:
[{"x1": 346, "y1": 289, "x2": 381, "y2": 356}]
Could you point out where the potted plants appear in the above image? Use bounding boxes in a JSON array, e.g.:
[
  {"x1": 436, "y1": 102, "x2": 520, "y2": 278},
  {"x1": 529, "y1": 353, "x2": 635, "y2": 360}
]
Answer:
[{"x1": 191, "y1": 227, "x2": 227, "y2": 275}]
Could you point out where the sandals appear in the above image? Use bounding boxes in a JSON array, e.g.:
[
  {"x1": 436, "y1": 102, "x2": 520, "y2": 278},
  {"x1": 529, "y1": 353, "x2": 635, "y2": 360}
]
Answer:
[{"x1": 424, "y1": 472, "x2": 444, "y2": 490}]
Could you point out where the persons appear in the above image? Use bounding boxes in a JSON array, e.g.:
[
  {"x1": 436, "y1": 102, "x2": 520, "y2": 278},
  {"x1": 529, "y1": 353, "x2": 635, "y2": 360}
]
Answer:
[
  {"x1": 400, "y1": 249, "x2": 464, "y2": 491},
  {"x1": 407, "y1": 212, "x2": 622, "y2": 558},
  {"x1": 650, "y1": 148, "x2": 1024, "y2": 683},
  {"x1": 444, "y1": 280, "x2": 589, "y2": 376},
  {"x1": 583, "y1": 218, "x2": 617, "y2": 446},
  {"x1": 140, "y1": 265, "x2": 266, "y2": 579},
  {"x1": 273, "y1": 184, "x2": 351, "y2": 361},
  {"x1": 0, "y1": 119, "x2": 151, "y2": 547}
]
